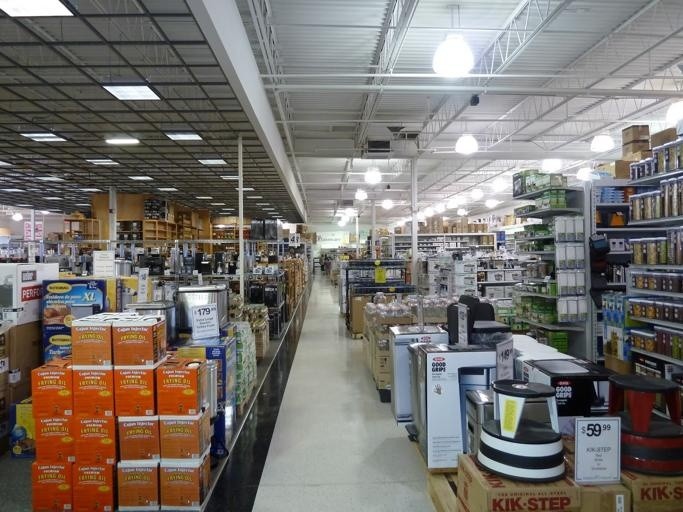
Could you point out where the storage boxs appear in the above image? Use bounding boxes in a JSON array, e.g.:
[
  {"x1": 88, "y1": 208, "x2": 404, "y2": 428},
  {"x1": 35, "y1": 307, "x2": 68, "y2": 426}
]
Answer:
[
  {"x1": 381, "y1": 320, "x2": 613, "y2": 477},
  {"x1": 457, "y1": 452, "x2": 683, "y2": 512},
  {"x1": 621, "y1": 124, "x2": 678, "y2": 147},
  {"x1": 0, "y1": 257, "x2": 308, "y2": 512}
]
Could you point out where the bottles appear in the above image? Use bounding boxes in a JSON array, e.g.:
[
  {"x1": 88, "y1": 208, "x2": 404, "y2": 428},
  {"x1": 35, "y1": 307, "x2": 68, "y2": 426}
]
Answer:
[{"x1": 366, "y1": 291, "x2": 498, "y2": 320}]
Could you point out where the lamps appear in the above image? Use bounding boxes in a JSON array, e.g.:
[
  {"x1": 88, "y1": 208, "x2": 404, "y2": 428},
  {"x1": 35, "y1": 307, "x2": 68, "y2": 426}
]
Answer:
[
  {"x1": 432, "y1": 5, "x2": 475, "y2": 78},
  {"x1": 590, "y1": 94, "x2": 616, "y2": 154},
  {"x1": 455, "y1": 120, "x2": 478, "y2": 156}
]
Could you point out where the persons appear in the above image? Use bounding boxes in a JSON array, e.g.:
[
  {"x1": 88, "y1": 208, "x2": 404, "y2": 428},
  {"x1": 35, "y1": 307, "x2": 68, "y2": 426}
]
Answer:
[{"x1": 319, "y1": 254, "x2": 331, "y2": 275}]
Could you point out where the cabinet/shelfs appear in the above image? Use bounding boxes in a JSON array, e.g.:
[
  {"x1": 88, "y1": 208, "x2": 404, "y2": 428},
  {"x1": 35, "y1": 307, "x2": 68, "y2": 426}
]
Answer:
[
  {"x1": 344, "y1": 227, "x2": 517, "y2": 342},
  {"x1": 505, "y1": 139, "x2": 681, "y2": 378},
  {"x1": 62, "y1": 193, "x2": 254, "y2": 251}
]
